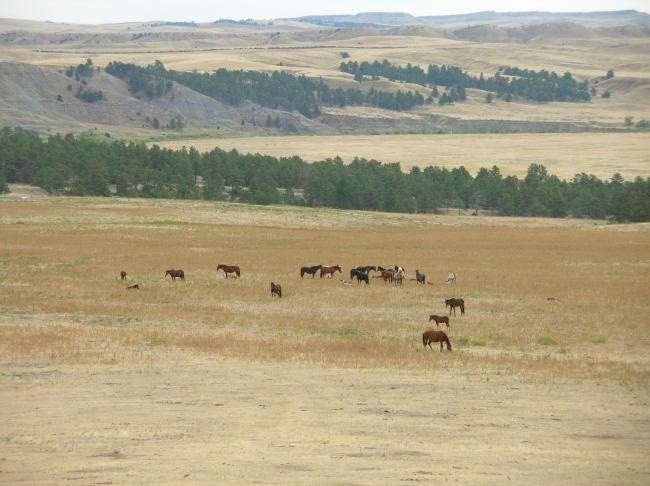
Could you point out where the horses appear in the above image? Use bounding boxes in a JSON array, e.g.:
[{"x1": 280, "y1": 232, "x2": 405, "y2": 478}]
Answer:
[
  {"x1": 300, "y1": 265, "x2": 342, "y2": 279},
  {"x1": 164, "y1": 269, "x2": 184, "y2": 281},
  {"x1": 447, "y1": 270, "x2": 456, "y2": 284},
  {"x1": 444, "y1": 298, "x2": 464, "y2": 316},
  {"x1": 350, "y1": 265, "x2": 426, "y2": 284},
  {"x1": 429, "y1": 314, "x2": 450, "y2": 328},
  {"x1": 271, "y1": 281, "x2": 282, "y2": 296},
  {"x1": 120, "y1": 270, "x2": 139, "y2": 290},
  {"x1": 217, "y1": 264, "x2": 240, "y2": 278},
  {"x1": 422, "y1": 331, "x2": 452, "y2": 352}
]
